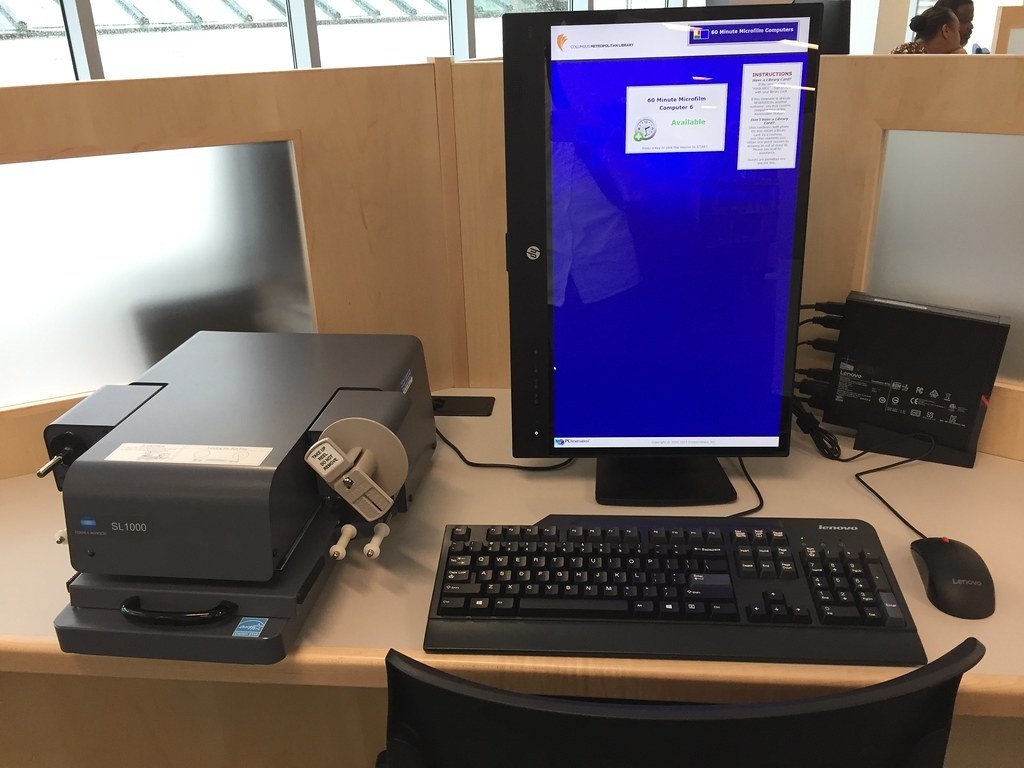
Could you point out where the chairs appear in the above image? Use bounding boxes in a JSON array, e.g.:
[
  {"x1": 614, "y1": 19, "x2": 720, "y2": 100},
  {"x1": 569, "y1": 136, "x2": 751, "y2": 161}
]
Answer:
[{"x1": 374, "y1": 638, "x2": 988, "y2": 768}]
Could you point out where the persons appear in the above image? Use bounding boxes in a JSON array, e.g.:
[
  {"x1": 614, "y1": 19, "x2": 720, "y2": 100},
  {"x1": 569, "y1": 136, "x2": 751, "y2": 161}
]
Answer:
[
  {"x1": 890, "y1": 7, "x2": 967, "y2": 54},
  {"x1": 935, "y1": 0, "x2": 973, "y2": 54}
]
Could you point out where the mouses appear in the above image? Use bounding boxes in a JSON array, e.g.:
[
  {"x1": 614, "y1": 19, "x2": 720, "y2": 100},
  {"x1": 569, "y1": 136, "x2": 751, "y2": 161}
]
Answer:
[{"x1": 911, "y1": 537, "x2": 996, "y2": 620}]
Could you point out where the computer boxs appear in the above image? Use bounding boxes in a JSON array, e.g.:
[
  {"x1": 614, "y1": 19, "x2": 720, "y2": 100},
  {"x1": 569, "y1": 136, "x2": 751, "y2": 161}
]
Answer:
[{"x1": 824, "y1": 290, "x2": 1011, "y2": 453}]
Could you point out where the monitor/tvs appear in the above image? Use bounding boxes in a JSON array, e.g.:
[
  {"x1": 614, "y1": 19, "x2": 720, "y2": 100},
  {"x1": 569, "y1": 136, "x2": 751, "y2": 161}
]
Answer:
[{"x1": 501, "y1": 3, "x2": 824, "y2": 506}]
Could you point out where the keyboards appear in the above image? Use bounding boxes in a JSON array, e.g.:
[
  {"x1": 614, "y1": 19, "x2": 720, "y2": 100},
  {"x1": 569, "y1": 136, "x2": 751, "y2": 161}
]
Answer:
[{"x1": 424, "y1": 513, "x2": 927, "y2": 666}]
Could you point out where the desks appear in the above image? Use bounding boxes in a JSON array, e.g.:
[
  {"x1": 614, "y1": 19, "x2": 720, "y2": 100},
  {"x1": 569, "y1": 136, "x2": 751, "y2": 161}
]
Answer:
[{"x1": 0, "y1": 389, "x2": 1024, "y2": 768}]
